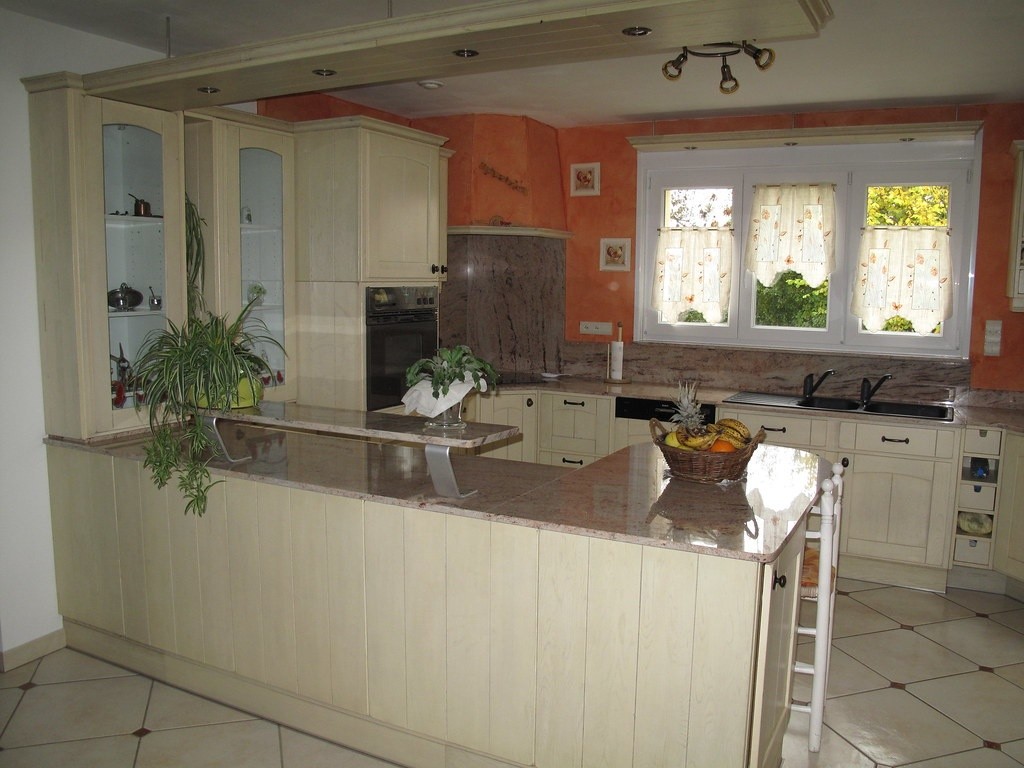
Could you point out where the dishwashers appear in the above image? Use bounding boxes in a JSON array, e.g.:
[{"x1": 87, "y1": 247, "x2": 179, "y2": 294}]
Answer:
[{"x1": 615, "y1": 396, "x2": 715, "y2": 456}]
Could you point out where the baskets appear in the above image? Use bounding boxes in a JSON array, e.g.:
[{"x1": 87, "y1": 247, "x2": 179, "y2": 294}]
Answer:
[{"x1": 648, "y1": 418, "x2": 767, "y2": 485}]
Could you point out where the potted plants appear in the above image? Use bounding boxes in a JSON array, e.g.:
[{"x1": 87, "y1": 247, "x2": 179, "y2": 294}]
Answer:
[
  {"x1": 405, "y1": 344, "x2": 500, "y2": 429},
  {"x1": 124, "y1": 281, "x2": 290, "y2": 518}
]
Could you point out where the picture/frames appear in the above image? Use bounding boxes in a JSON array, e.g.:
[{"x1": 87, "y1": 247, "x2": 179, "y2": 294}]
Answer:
[
  {"x1": 569, "y1": 162, "x2": 601, "y2": 197},
  {"x1": 599, "y1": 238, "x2": 632, "y2": 272}
]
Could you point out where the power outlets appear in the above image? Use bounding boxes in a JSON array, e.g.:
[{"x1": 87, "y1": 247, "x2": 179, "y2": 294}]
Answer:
[
  {"x1": 984, "y1": 319, "x2": 1003, "y2": 358},
  {"x1": 579, "y1": 321, "x2": 613, "y2": 336}
]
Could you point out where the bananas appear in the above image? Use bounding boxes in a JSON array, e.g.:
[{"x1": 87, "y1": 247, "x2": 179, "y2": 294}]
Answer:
[
  {"x1": 705, "y1": 418, "x2": 752, "y2": 449},
  {"x1": 675, "y1": 421, "x2": 722, "y2": 449}
]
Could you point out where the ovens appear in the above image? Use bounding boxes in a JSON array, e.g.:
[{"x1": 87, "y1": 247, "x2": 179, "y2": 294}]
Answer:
[{"x1": 367, "y1": 287, "x2": 439, "y2": 412}]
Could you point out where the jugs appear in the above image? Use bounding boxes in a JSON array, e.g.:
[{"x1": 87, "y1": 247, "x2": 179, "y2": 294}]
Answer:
[{"x1": 241, "y1": 280, "x2": 264, "y2": 306}]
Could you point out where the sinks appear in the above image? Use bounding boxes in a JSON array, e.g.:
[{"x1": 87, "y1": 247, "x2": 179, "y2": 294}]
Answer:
[
  {"x1": 861, "y1": 400, "x2": 953, "y2": 420},
  {"x1": 792, "y1": 397, "x2": 863, "y2": 413}
]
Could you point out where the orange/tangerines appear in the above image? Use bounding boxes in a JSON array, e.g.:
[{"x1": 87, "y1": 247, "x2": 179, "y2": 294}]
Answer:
[{"x1": 710, "y1": 439, "x2": 736, "y2": 452}]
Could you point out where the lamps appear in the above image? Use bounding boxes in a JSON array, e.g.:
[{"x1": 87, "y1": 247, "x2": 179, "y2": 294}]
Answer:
[
  {"x1": 719, "y1": 56, "x2": 739, "y2": 95},
  {"x1": 742, "y1": 44, "x2": 776, "y2": 71},
  {"x1": 662, "y1": 49, "x2": 688, "y2": 82}
]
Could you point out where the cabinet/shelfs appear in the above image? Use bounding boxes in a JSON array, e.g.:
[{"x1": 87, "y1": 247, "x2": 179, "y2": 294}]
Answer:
[
  {"x1": 294, "y1": 114, "x2": 449, "y2": 281},
  {"x1": 440, "y1": 148, "x2": 458, "y2": 283},
  {"x1": 718, "y1": 407, "x2": 835, "y2": 553},
  {"x1": 538, "y1": 390, "x2": 613, "y2": 469},
  {"x1": 830, "y1": 417, "x2": 961, "y2": 595},
  {"x1": 743, "y1": 517, "x2": 806, "y2": 768},
  {"x1": 478, "y1": 389, "x2": 537, "y2": 465},
  {"x1": 368, "y1": 392, "x2": 478, "y2": 456},
  {"x1": 21, "y1": 70, "x2": 191, "y2": 446},
  {"x1": 992, "y1": 429, "x2": 1024, "y2": 604},
  {"x1": 181, "y1": 120, "x2": 299, "y2": 405},
  {"x1": 945, "y1": 424, "x2": 1007, "y2": 597}
]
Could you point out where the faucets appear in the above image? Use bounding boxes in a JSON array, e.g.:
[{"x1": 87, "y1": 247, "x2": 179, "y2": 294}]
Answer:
[
  {"x1": 861, "y1": 374, "x2": 893, "y2": 406},
  {"x1": 803, "y1": 368, "x2": 835, "y2": 399}
]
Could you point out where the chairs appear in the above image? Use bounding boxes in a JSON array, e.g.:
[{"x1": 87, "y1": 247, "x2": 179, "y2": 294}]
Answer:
[{"x1": 788, "y1": 461, "x2": 845, "y2": 755}]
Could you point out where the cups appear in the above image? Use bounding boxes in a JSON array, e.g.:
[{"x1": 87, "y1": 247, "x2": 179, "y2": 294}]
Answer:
[
  {"x1": 149, "y1": 296, "x2": 161, "y2": 310},
  {"x1": 241, "y1": 207, "x2": 251, "y2": 224}
]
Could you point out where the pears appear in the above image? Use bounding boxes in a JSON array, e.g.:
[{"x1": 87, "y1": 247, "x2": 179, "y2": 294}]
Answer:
[{"x1": 664, "y1": 428, "x2": 694, "y2": 450}]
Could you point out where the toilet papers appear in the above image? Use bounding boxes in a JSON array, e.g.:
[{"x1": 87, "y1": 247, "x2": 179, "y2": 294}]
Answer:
[{"x1": 610, "y1": 341, "x2": 624, "y2": 380}]
